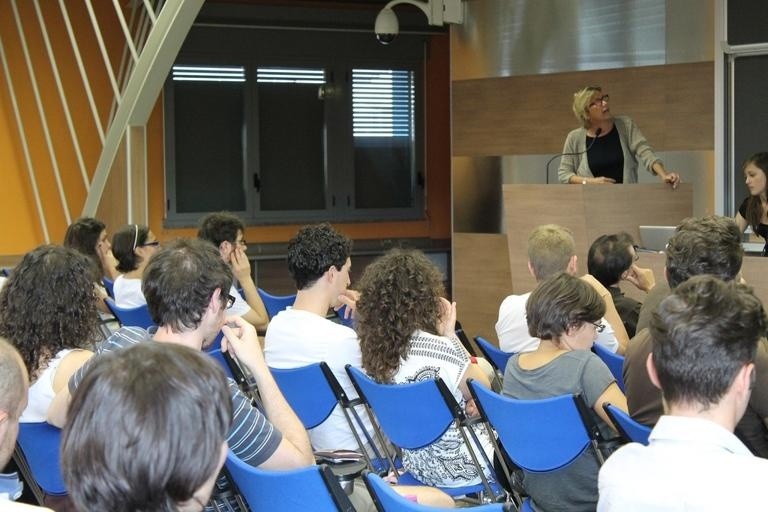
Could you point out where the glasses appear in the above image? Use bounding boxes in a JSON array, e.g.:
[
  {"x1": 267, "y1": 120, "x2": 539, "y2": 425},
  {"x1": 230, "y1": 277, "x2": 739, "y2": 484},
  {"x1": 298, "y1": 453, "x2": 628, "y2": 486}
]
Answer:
[
  {"x1": 225, "y1": 295, "x2": 236, "y2": 308},
  {"x1": 591, "y1": 322, "x2": 605, "y2": 332},
  {"x1": 142, "y1": 241, "x2": 159, "y2": 248},
  {"x1": 588, "y1": 95, "x2": 608, "y2": 106}
]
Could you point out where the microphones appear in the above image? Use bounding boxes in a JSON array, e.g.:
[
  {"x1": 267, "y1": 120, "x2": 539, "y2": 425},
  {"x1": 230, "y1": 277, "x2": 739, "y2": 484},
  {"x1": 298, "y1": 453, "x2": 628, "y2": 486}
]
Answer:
[{"x1": 546, "y1": 127, "x2": 602, "y2": 184}]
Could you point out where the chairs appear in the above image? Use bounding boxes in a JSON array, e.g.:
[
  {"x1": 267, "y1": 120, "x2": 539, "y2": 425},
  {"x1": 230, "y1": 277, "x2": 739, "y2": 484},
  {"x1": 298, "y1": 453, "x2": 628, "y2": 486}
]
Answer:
[{"x1": 0, "y1": 268, "x2": 653, "y2": 512}]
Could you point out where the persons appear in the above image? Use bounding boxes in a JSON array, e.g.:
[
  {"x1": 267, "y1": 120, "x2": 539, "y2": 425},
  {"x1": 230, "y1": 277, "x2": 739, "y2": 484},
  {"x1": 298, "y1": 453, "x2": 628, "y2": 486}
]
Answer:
[
  {"x1": 263, "y1": 222, "x2": 395, "y2": 459},
  {"x1": 494, "y1": 226, "x2": 630, "y2": 357},
  {"x1": 585, "y1": 230, "x2": 656, "y2": 337},
  {"x1": 57, "y1": 341, "x2": 235, "y2": 511},
  {"x1": 66, "y1": 219, "x2": 161, "y2": 308},
  {"x1": 624, "y1": 215, "x2": 768, "y2": 455},
  {"x1": 0, "y1": 335, "x2": 54, "y2": 511},
  {"x1": 197, "y1": 214, "x2": 270, "y2": 333},
  {"x1": 361, "y1": 248, "x2": 501, "y2": 489},
  {"x1": 491, "y1": 273, "x2": 627, "y2": 512},
  {"x1": 558, "y1": 86, "x2": 681, "y2": 191},
  {"x1": 1, "y1": 247, "x2": 106, "y2": 428},
  {"x1": 596, "y1": 275, "x2": 767, "y2": 511},
  {"x1": 46, "y1": 234, "x2": 316, "y2": 510},
  {"x1": 734, "y1": 151, "x2": 768, "y2": 258}
]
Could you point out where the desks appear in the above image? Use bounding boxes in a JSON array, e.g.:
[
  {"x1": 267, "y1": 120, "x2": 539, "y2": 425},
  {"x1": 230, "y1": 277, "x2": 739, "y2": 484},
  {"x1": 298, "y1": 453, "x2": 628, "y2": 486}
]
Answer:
[{"x1": 621, "y1": 251, "x2": 767, "y2": 315}]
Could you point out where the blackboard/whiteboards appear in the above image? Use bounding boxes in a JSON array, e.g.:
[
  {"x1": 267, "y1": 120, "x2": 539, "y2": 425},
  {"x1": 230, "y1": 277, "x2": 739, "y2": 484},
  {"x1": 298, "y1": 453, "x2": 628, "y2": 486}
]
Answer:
[
  {"x1": 722, "y1": 0, "x2": 768, "y2": 55},
  {"x1": 730, "y1": 53, "x2": 768, "y2": 235}
]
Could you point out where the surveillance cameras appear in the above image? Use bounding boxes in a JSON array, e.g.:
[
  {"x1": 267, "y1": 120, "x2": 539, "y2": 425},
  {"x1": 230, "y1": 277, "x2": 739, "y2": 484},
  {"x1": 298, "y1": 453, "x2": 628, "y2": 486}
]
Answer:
[{"x1": 375, "y1": 7, "x2": 399, "y2": 47}]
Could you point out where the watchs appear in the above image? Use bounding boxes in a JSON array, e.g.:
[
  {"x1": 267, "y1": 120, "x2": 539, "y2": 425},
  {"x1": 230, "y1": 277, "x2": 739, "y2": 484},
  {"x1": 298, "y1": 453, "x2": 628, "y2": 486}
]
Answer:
[{"x1": 582, "y1": 177, "x2": 587, "y2": 185}]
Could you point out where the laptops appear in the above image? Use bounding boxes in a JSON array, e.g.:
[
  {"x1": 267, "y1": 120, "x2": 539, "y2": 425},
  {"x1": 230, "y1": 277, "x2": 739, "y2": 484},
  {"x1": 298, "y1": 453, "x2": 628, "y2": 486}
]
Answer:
[{"x1": 639, "y1": 226, "x2": 677, "y2": 252}]
